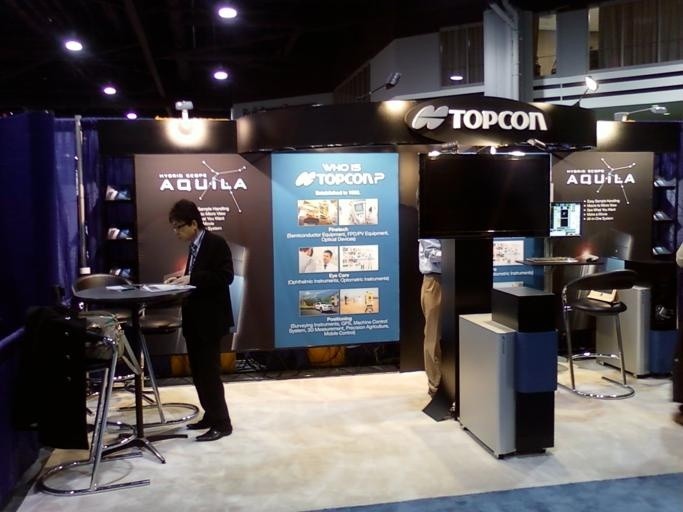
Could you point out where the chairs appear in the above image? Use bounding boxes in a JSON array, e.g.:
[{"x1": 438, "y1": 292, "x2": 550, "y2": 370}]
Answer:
[
  {"x1": 72, "y1": 272, "x2": 199, "y2": 434},
  {"x1": 27, "y1": 305, "x2": 152, "y2": 498},
  {"x1": 557, "y1": 268, "x2": 639, "y2": 400}
]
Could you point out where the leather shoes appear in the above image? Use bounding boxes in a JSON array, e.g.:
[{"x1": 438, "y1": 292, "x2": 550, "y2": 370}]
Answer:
[
  {"x1": 194, "y1": 426, "x2": 233, "y2": 443},
  {"x1": 184, "y1": 418, "x2": 211, "y2": 430}
]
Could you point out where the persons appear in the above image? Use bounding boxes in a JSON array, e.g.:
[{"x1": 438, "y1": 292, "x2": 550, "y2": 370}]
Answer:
[
  {"x1": 316, "y1": 249, "x2": 337, "y2": 270},
  {"x1": 299, "y1": 247, "x2": 317, "y2": 274},
  {"x1": 675, "y1": 243, "x2": 683, "y2": 417},
  {"x1": 415, "y1": 237, "x2": 446, "y2": 399},
  {"x1": 161, "y1": 197, "x2": 233, "y2": 443}
]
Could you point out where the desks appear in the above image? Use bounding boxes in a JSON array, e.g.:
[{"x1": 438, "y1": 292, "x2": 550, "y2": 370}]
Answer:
[
  {"x1": 73, "y1": 283, "x2": 194, "y2": 463},
  {"x1": 515, "y1": 259, "x2": 604, "y2": 372}
]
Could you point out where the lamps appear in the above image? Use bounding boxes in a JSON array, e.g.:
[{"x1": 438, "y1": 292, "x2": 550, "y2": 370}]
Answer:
[
  {"x1": 355, "y1": 71, "x2": 401, "y2": 100},
  {"x1": 614, "y1": 105, "x2": 668, "y2": 121},
  {"x1": 572, "y1": 76, "x2": 599, "y2": 107}
]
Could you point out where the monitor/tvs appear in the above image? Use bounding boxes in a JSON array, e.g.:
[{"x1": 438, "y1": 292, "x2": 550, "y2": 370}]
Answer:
[
  {"x1": 417, "y1": 151, "x2": 551, "y2": 240},
  {"x1": 549, "y1": 202, "x2": 581, "y2": 239}
]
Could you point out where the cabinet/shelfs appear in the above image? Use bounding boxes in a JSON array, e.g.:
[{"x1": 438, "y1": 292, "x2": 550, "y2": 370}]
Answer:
[{"x1": 595, "y1": 119, "x2": 683, "y2": 260}]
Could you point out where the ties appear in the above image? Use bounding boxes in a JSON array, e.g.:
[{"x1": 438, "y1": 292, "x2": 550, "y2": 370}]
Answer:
[{"x1": 186, "y1": 243, "x2": 198, "y2": 277}]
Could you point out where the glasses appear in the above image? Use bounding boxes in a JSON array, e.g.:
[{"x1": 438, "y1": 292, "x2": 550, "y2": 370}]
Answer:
[{"x1": 171, "y1": 222, "x2": 189, "y2": 233}]
